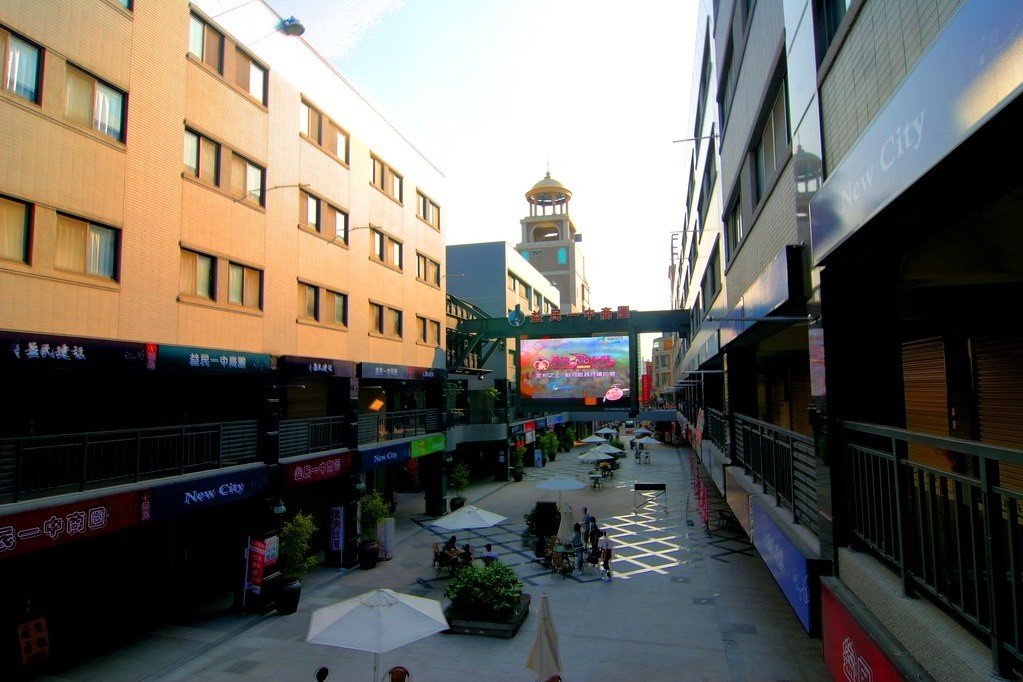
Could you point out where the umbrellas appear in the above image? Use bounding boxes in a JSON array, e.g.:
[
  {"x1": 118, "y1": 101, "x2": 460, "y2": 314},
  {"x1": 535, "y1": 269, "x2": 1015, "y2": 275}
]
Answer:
[
  {"x1": 632, "y1": 426, "x2": 652, "y2": 434},
  {"x1": 577, "y1": 426, "x2": 622, "y2": 462},
  {"x1": 536, "y1": 472, "x2": 586, "y2": 506},
  {"x1": 306, "y1": 583, "x2": 450, "y2": 682},
  {"x1": 430, "y1": 502, "x2": 508, "y2": 545}
]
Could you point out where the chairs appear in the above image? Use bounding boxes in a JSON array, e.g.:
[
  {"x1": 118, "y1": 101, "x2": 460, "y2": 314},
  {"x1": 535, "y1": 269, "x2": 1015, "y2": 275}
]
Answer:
[
  {"x1": 431, "y1": 542, "x2": 497, "y2": 575},
  {"x1": 381, "y1": 665, "x2": 410, "y2": 682},
  {"x1": 530, "y1": 539, "x2": 599, "y2": 579}
]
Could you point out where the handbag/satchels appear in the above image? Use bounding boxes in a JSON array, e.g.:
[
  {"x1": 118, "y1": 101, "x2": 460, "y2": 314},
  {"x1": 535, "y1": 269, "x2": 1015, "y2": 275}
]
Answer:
[{"x1": 603, "y1": 549, "x2": 612, "y2": 560}]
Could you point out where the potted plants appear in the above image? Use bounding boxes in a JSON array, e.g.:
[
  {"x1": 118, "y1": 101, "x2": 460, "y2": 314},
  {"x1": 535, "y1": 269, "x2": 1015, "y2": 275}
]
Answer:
[
  {"x1": 538, "y1": 432, "x2": 560, "y2": 466},
  {"x1": 512, "y1": 445, "x2": 528, "y2": 482},
  {"x1": 269, "y1": 511, "x2": 319, "y2": 615},
  {"x1": 350, "y1": 488, "x2": 389, "y2": 570},
  {"x1": 560, "y1": 427, "x2": 576, "y2": 453},
  {"x1": 449, "y1": 460, "x2": 469, "y2": 508}
]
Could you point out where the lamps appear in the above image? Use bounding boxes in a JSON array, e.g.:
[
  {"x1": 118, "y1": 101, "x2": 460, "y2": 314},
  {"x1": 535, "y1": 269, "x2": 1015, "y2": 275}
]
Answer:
[{"x1": 286, "y1": 17, "x2": 306, "y2": 36}]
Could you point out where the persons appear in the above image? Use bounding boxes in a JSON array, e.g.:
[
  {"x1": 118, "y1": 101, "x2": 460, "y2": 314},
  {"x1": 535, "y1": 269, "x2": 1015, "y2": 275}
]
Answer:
[
  {"x1": 570, "y1": 507, "x2": 613, "y2": 582},
  {"x1": 632, "y1": 435, "x2": 661, "y2": 444},
  {"x1": 440, "y1": 536, "x2": 499, "y2": 580}
]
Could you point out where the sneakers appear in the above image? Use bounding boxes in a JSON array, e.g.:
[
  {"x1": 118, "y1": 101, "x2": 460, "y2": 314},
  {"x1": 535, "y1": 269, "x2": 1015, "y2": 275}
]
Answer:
[
  {"x1": 600, "y1": 576, "x2": 605, "y2": 579},
  {"x1": 576, "y1": 570, "x2": 585, "y2": 575},
  {"x1": 604, "y1": 578, "x2": 612, "y2": 582}
]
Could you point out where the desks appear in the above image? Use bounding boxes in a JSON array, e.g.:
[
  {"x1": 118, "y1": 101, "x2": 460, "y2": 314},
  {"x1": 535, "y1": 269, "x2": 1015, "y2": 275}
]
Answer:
[
  {"x1": 553, "y1": 546, "x2": 577, "y2": 569},
  {"x1": 590, "y1": 474, "x2": 603, "y2": 487}
]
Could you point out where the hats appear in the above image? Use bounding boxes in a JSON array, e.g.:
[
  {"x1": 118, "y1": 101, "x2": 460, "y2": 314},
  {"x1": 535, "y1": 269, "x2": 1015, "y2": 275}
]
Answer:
[
  {"x1": 574, "y1": 523, "x2": 581, "y2": 531},
  {"x1": 485, "y1": 543, "x2": 491, "y2": 548}
]
